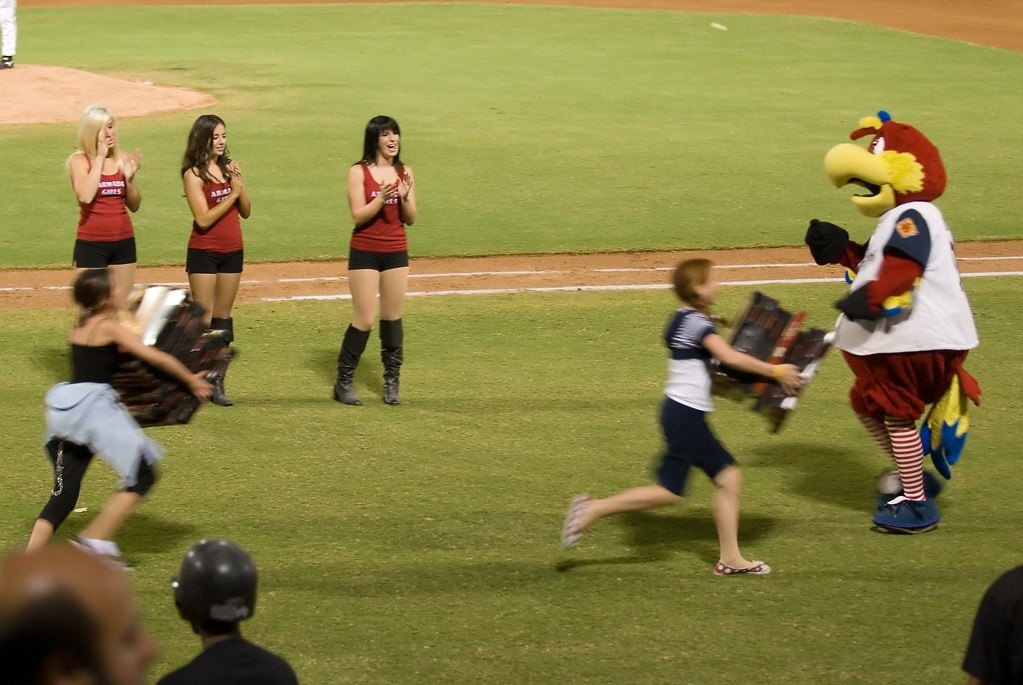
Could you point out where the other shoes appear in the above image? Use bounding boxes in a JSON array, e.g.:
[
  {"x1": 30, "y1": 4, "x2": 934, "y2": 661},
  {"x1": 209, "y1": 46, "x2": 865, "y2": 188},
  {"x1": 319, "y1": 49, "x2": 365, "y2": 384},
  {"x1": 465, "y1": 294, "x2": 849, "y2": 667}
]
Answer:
[
  {"x1": 560, "y1": 495, "x2": 594, "y2": 552},
  {"x1": 714, "y1": 558, "x2": 772, "y2": 577},
  {"x1": 67, "y1": 536, "x2": 136, "y2": 574}
]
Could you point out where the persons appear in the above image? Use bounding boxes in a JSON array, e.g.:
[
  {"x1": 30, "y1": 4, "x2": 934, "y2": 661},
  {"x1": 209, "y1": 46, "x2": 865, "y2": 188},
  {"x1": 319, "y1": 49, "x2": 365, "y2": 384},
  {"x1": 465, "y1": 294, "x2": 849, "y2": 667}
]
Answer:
[
  {"x1": 561, "y1": 259, "x2": 807, "y2": 576},
  {"x1": 68, "y1": 104, "x2": 142, "y2": 311},
  {"x1": 959, "y1": 564, "x2": 1023, "y2": 685},
  {"x1": 333, "y1": 116, "x2": 417, "y2": 405},
  {"x1": 154, "y1": 540, "x2": 300, "y2": 685},
  {"x1": 24, "y1": 268, "x2": 214, "y2": 569},
  {"x1": 0, "y1": 542, "x2": 164, "y2": 685},
  {"x1": 180, "y1": 114, "x2": 251, "y2": 406},
  {"x1": 0, "y1": 0, "x2": 18, "y2": 69}
]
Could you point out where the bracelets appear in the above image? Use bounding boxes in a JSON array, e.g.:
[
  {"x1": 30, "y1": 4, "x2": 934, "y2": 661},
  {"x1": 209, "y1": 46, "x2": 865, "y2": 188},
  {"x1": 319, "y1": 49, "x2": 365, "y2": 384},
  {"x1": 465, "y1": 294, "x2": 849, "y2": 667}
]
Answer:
[{"x1": 772, "y1": 364, "x2": 786, "y2": 378}]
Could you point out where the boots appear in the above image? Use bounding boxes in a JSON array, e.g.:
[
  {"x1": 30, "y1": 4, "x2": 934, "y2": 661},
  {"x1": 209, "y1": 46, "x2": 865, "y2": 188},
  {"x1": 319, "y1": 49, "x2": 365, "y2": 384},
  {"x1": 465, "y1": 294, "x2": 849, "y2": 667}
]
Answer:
[
  {"x1": 206, "y1": 316, "x2": 233, "y2": 406},
  {"x1": 379, "y1": 318, "x2": 403, "y2": 405},
  {"x1": 334, "y1": 323, "x2": 364, "y2": 406}
]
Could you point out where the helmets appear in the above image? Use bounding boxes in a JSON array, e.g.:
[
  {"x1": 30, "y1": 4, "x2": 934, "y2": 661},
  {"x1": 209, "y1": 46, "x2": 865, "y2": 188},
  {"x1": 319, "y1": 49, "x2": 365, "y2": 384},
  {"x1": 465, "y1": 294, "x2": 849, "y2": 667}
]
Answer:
[{"x1": 174, "y1": 539, "x2": 258, "y2": 621}]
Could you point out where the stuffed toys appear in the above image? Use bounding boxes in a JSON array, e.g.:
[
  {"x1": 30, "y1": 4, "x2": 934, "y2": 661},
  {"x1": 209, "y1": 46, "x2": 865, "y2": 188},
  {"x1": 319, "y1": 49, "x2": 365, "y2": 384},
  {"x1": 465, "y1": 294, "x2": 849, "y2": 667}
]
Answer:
[{"x1": 805, "y1": 111, "x2": 982, "y2": 535}]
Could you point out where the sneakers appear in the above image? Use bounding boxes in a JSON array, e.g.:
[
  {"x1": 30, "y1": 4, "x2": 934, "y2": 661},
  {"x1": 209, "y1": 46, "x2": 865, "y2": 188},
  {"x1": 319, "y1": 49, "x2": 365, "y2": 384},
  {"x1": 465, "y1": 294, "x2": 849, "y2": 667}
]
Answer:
[{"x1": 0, "y1": 56, "x2": 13, "y2": 69}]
